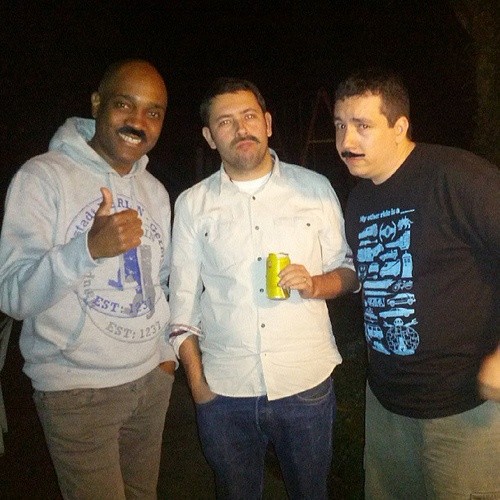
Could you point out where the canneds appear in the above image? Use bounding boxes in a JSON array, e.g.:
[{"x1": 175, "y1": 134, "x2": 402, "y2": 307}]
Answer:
[{"x1": 265, "y1": 251, "x2": 292, "y2": 301}]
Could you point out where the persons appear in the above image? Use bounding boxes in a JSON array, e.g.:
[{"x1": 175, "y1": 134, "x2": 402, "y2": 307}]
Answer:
[
  {"x1": 0, "y1": 51, "x2": 180, "y2": 499},
  {"x1": 332, "y1": 71, "x2": 499, "y2": 499},
  {"x1": 165, "y1": 78, "x2": 364, "y2": 499}
]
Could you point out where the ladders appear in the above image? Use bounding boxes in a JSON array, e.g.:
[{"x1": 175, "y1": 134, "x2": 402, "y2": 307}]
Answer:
[{"x1": 298, "y1": 84, "x2": 335, "y2": 166}]
